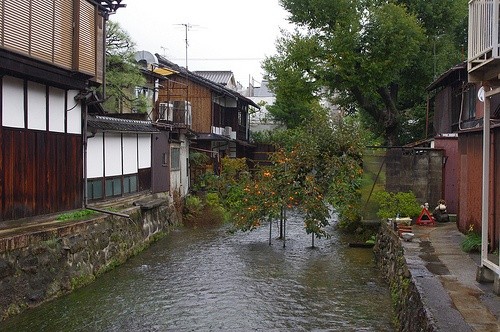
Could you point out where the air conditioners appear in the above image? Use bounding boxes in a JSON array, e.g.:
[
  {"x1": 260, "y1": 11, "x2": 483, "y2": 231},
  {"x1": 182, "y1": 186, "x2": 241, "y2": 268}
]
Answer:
[
  {"x1": 159, "y1": 103, "x2": 173, "y2": 121},
  {"x1": 225, "y1": 126, "x2": 233, "y2": 138}
]
